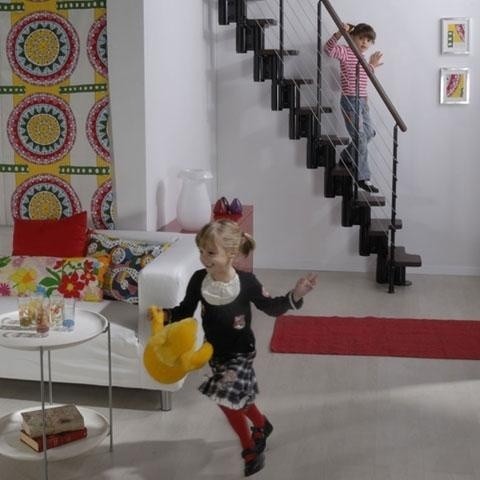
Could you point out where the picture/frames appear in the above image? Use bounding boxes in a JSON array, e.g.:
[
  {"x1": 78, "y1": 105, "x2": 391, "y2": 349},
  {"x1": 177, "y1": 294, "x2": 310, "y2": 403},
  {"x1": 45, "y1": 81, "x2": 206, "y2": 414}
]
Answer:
[{"x1": 438, "y1": 16, "x2": 473, "y2": 106}]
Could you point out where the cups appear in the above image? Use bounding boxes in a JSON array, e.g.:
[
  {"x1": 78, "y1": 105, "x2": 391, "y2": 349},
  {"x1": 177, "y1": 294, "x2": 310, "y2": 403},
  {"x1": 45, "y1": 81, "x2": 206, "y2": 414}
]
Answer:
[{"x1": 17, "y1": 293, "x2": 77, "y2": 338}]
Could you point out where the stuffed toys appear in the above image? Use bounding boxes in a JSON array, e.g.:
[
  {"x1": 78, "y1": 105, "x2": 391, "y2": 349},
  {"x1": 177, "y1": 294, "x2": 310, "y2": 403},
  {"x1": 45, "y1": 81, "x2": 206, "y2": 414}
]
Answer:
[{"x1": 144, "y1": 304, "x2": 215, "y2": 385}]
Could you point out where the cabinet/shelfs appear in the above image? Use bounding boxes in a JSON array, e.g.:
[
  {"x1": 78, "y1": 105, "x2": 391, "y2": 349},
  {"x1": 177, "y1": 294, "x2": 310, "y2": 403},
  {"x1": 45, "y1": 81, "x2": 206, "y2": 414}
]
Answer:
[{"x1": 0, "y1": 304, "x2": 114, "y2": 480}]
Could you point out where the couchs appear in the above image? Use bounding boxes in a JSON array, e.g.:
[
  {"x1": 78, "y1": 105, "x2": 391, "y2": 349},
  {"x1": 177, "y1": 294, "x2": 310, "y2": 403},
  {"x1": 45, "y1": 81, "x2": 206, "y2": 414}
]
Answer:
[{"x1": 0, "y1": 224, "x2": 206, "y2": 412}]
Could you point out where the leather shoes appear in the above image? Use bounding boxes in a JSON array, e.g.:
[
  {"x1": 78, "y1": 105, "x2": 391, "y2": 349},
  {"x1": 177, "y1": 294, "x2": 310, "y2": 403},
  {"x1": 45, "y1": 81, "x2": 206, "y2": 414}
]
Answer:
[
  {"x1": 242, "y1": 448, "x2": 264, "y2": 476},
  {"x1": 354, "y1": 180, "x2": 378, "y2": 193},
  {"x1": 339, "y1": 158, "x2": 352, "y2": 169},
  {"x1": 250, "y1": 416, "x2": 272, "y2": 453}
]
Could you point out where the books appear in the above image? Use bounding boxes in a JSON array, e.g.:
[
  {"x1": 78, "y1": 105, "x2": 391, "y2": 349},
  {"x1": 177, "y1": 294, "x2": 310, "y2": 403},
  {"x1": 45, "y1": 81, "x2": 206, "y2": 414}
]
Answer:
[
  {"x1": 19, "y1": 428, "x2": 87, "y2": 452},
  {"x1": 20, "y1": 402, "x2": 85, "y2": 437}
]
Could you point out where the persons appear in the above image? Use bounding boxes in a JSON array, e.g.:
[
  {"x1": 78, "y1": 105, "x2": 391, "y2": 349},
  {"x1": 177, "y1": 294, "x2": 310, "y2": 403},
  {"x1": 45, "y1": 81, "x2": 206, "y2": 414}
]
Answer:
[
  {"x1": 323, "y1": 23, "x2": 385, "y2": 191},
  {"x1": 145, "y1": 218, "x2": 319, "y2": 474}
]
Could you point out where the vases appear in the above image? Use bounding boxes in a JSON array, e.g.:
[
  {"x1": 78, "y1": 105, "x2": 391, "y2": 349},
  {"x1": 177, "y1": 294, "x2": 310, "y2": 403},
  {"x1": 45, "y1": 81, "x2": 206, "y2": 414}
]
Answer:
[{"x1": 175, "y1": 167, "x2": 214, "y2": 232}]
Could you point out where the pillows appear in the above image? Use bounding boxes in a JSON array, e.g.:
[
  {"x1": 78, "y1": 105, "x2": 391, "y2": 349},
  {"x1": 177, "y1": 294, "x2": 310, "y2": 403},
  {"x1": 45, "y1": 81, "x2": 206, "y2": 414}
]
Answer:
[{"x1": 0, "y1": 209, "x2": 172, "y2": 307}]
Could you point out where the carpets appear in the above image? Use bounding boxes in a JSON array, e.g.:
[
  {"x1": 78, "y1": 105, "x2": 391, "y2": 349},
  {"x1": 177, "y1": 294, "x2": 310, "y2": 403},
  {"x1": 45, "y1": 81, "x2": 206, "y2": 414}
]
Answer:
[{"x1": 268, "y1": 314, "x2": 480, "y2": 360}]
